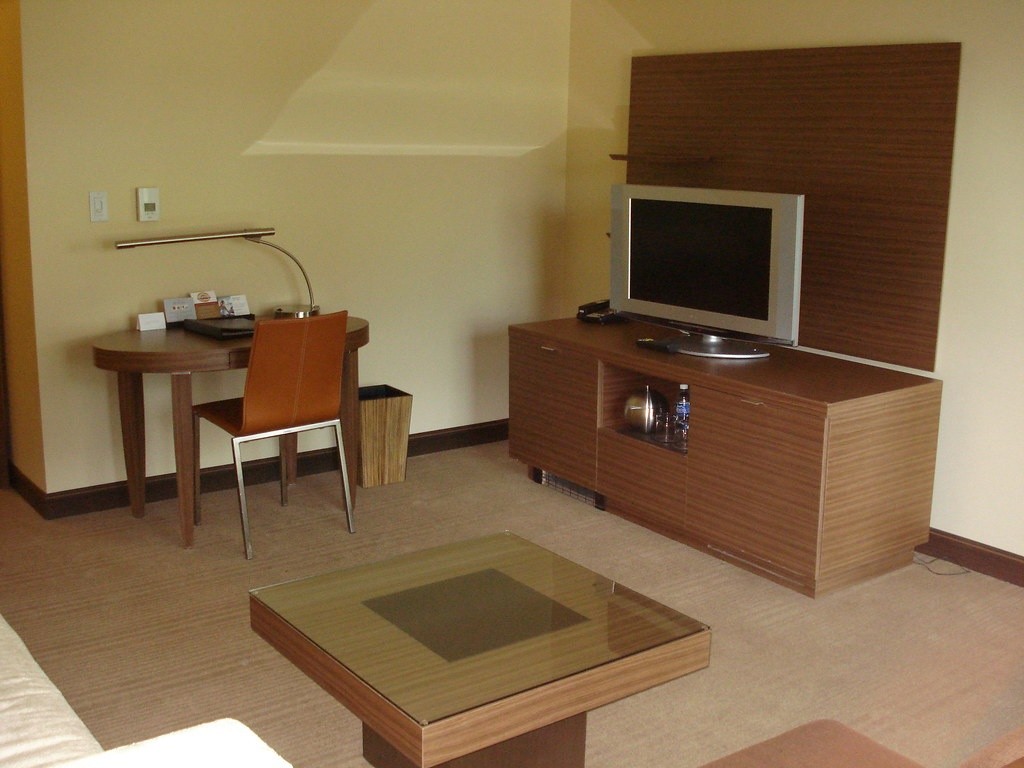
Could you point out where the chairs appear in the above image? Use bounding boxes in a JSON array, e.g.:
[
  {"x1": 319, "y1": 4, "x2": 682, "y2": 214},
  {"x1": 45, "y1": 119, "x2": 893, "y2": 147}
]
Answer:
[
  {"x1": 192, "y1": 310, "x2": 354, "y2": 559},
  {"x1": 702, "y1": 718, "x2": 1024, "y2": 768}
]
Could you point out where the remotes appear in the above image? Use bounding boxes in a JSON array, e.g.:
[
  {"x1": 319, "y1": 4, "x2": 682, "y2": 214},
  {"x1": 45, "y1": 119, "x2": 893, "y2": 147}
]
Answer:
[{"x1": 637, "y1": 338, "x2": 673, "y2": 354}]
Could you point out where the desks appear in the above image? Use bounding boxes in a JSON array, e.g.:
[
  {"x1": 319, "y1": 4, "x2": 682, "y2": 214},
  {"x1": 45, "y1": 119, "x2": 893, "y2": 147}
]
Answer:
[
  {"x1": 91, "y1": 311, "x2": 370, "y2": 550},
  {"x1": 251, "y1": 530, "x2": 711, "y2": 768}
]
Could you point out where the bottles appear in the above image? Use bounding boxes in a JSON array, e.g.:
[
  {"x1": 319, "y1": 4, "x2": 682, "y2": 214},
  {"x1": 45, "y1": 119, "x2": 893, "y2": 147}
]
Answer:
[{"x1": 676, "y1": 384, "x2": 690, "y2": 439}]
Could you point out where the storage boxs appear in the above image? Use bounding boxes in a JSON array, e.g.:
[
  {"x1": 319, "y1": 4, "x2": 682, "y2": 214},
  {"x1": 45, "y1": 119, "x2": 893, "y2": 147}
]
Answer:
[{"x1": 357, "y1": 384, "x2": 413, "y2": 489}]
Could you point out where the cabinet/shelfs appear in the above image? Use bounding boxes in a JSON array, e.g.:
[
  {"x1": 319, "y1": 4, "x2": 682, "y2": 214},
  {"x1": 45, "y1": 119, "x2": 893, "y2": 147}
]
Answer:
[
  {"x1": 687, "y1": 383, "x2": 829, "y2": 581},
  {"x1": 507, "y1": 324, "x2": 600, "y2": 492}
]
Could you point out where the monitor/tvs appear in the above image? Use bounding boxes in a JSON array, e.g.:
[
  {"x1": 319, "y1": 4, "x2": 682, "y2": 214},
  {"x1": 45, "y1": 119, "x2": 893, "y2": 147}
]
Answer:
[{"x1": 610, "y1": 182, "x2": 806, "y2": 359}]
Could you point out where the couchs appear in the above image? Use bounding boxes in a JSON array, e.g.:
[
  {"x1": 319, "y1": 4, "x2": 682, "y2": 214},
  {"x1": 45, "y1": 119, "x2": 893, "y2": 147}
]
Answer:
[{"x1": 0, "y1": 614, "x2": 294, "y2": 767}]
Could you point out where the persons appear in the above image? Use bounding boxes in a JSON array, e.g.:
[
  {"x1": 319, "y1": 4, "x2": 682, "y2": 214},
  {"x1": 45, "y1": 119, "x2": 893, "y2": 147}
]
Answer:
[{"x1": 219, "y1": 300, "x2": 229, "y2": 315}]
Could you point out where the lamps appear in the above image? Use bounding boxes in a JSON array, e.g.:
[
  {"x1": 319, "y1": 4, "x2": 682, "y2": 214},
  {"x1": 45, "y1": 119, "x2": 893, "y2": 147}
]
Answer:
[{"x1": 116, "y1": 228, "x2": 320, "y2": 319}]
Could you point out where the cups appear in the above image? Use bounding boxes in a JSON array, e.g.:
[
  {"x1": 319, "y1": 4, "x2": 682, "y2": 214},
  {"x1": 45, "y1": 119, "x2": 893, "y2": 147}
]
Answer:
[{"x1": 655, "y1": 407, "x2": 676, "y2": 443}]
